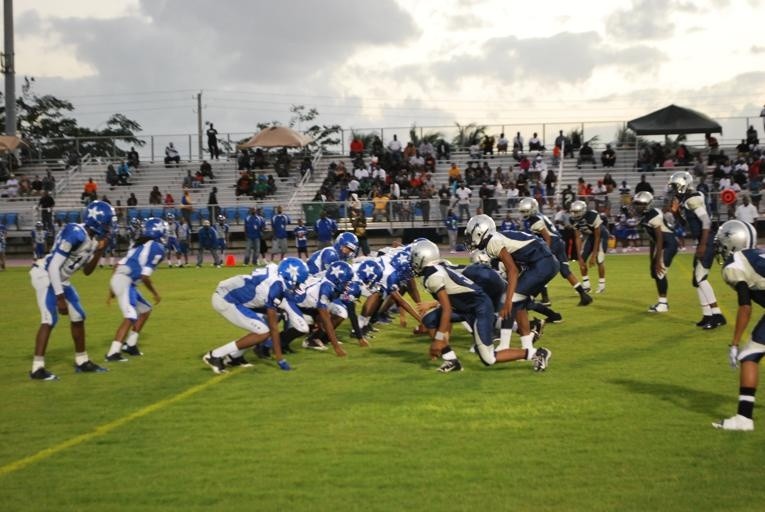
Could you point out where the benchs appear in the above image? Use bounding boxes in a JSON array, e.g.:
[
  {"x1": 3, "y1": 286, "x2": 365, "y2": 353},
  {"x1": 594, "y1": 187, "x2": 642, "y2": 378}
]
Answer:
[{"x1": 1, "y1": 146, "x2": 764, "y2": 236}]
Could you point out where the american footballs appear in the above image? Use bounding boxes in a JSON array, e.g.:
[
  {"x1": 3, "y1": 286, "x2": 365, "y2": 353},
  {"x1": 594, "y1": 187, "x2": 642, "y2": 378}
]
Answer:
[{"x1": 414, "y1": 323, "x2": 427, "y2": 334}]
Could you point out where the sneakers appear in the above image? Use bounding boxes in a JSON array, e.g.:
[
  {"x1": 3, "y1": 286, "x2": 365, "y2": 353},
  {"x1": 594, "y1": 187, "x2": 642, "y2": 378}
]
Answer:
[
  {"x1": 533, "y1": 347, "x2": 552, "y2": 372},
  {"x1": 75, "y1": 361, "x2": 108, "y2": 373},
  {"x1": 609, "y1": 245, "x2": 699, "y2": 252},
  {"x1": 30, "y1": 368, "x2": 60, "y2": 381},
  {"x1": 517, "y1": 283, "x2": 606, "y2": 342},
  {"x1": 203, "y1": 350, "x2": 227, "y2": 374},
  {"x1": 99, "y1": 261, "x2": 271, "y2": 269},
  {"x1": 105, "y1": 354, "x2": 128, "y2": 363},
  {"x1": 122, "y1": 342, "x2": 144, "y2": 357},
  {"x1": 648, "y1": 301, "x2": 669, "y2": 313},
  {"x1": 223, "y1": 351, "x2": 255, "y2": 369},
  {"x1": 436, "y1": 358, "x2": 466, "y2": 373},
  {"x1": 255, "y1": 307, "x2": 400, "y2": 357},
  {"x1": 696, "y1": 313, "x2": 726, "y2": 330},
  {"x1": 712, "y1": 413, "x2": 755, "y2": 431}
]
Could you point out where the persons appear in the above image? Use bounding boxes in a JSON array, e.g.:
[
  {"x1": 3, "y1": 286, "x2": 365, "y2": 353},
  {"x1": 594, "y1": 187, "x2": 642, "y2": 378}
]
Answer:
[
  {"x1": 104, "y1": 217, "x2": 171, "y2": 362},
  {"x1": 413, "y1": 197, "x2": 609, "y2": 372},
  {"x1": 0, "y1": 107, "x2": 765, "y2": 270},
  {"x1": 201, "y1": 230, "x2": 426, "y2": 374},
  {"x1": 28, "y1": 200, "x2": 117, "y2": 385},
  {"x1": 633, "y1": 171, "x2": 728, "y2": 330},
  {"x1": 713, "y1": 218, "x2": 765, "y2": 432}
]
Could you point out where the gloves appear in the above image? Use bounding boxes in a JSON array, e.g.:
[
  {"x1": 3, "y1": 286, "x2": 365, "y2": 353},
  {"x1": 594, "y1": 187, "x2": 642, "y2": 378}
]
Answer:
[{"x1": 278, "y1": 359, "x2": 296, "y2": 371}]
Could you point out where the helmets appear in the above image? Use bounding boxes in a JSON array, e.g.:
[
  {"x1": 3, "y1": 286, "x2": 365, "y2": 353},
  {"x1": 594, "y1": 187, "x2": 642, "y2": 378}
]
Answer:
[
  {"x1": 35, "y1": 221, "x2": 43, "y2": 226},
  {"x1": 569, "y1": 201, "x2": 588, "y2": 220},
  {"x1": 391, "y1": 251, "x2": 411, "y2": 274},
  {"x1": 666, "y1": 170, "x2": 696, "y2": 195},
  {"x1": 142, "y1": 216, "x2": 169, "y2": 241},
  {"x1": 326, "y1": 262, "x2": 354, "y2": 293},
  {"x1": 82, "y1": 199, "x2": 118, "y2": 234},
  {"x1": 519, "y1": 196, "x2": 540, "y2": 218},
  {"x1": 632, "y1": 191, "x2": 655, "y2": 214},
  {"x1": 167, "y1": 213, "x2": 228, "y2": 227},
  {"x1": 411, "y1": 240, "x2": 440, "y2": 274},
  {"x1": 356, "y1": 260, "x2": 383, "y2": 290},
  {"x1": 276, "y1": 255, "x2": 309, "y2": 290},
  {"x1": 335, "y1": 231, "x2": 361, "y2": 261},
  {"x1": 464, "y1": 214, "x2": 497, "y2": 248},
  {"x1": 714, "y1": 218, "x2": 758, "y2": 258}
]
